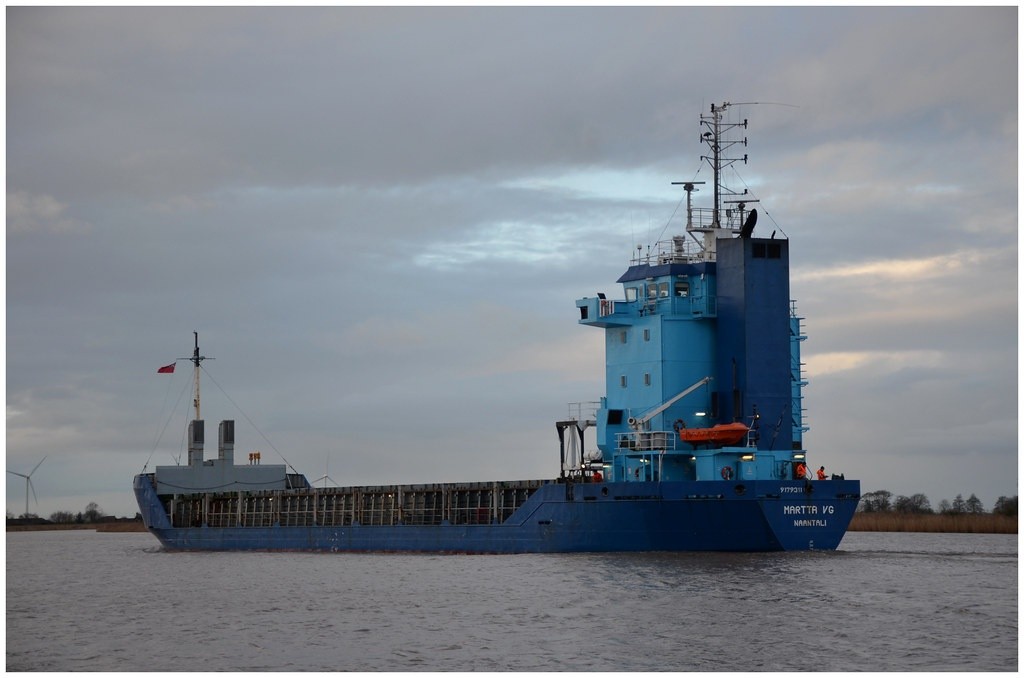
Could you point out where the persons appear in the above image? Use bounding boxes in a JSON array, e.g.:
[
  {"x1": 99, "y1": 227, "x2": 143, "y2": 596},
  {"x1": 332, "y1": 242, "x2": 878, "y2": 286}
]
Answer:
[
  {"x1": 796, "y1": 462, "x2": 807, "y2": 480},
  {"x1": 816, "y1": 466, "x2": 826, "y2": 480},
  {"x1": 592, "y1": 470, "x2": 602, "y2": 483}
]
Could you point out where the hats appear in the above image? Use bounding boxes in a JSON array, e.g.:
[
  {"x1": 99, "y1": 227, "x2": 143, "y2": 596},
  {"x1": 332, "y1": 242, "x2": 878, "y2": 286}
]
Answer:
[{"x1": 802, "y1": 461, "x2": 806, "y2": 467}]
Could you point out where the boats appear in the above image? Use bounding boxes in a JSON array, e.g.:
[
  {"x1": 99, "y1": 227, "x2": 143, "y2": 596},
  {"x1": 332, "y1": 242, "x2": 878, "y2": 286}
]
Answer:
[
  {"x1": 130, "y1": 102, "x2": 861, "y2": 555},
  {"x1": 679, "y1": 422, "x2": 750, "y2": 446}
]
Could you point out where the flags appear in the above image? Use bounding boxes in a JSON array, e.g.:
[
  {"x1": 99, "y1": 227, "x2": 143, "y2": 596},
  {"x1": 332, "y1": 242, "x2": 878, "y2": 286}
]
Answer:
[{"x1": 157, "y1": 363, "x2": 176, "y2": 373}]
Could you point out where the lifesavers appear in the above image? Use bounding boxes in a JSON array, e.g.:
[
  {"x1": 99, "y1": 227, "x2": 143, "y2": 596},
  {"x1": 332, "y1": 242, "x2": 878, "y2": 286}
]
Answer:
[{"x1": 722, "y1": 466, "x2": 734, "y2": 480}]
